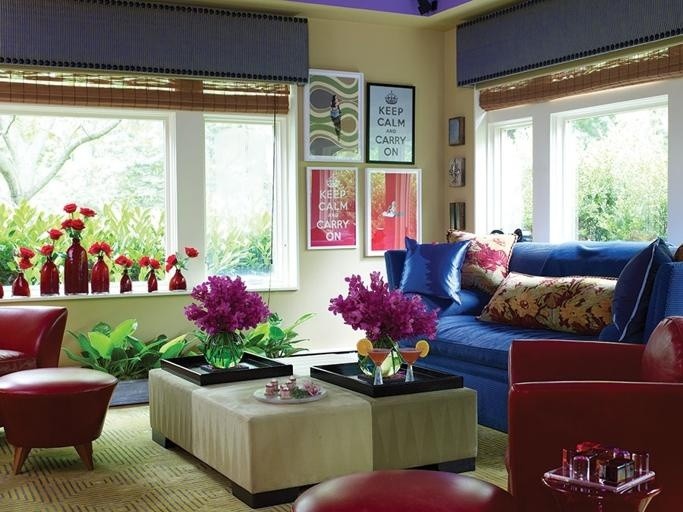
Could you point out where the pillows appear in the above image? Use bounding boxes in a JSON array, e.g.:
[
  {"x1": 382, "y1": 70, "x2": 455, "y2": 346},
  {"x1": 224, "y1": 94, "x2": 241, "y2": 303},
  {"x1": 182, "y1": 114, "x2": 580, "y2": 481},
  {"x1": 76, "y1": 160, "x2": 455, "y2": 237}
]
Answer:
[
  {"x1": 612, "y1": 236, "x2": 672, "y2": 343},
  {"x1": 447, "y1": 228, "x2": 517, "y2": 291},
  {"x1": 399, "y1": 233, "x2": 469, "y2": 306},
  {"x1": 476, "y1": 270, "x2": 615, "y2": 334}
]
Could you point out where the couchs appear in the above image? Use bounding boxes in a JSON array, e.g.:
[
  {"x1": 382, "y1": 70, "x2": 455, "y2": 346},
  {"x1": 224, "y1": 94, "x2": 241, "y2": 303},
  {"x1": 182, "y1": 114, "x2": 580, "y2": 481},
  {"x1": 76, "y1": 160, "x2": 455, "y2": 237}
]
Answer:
[{"x1": 384, "y1": 244, "x2": 683, "y2": 434}]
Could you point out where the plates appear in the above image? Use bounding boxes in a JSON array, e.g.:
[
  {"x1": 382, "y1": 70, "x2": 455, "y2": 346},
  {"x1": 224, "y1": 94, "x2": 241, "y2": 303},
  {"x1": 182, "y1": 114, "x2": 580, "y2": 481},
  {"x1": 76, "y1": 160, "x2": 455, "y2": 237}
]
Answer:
[
  {"x1": 160, "y1": 350, "x2": 294, "y2": 386},
  {"x1": 309, "y1": 361, "x2": 464, "y2": 398},
  {"x1": 253, "y1": 384, "x2": 328, "y2": 404}
]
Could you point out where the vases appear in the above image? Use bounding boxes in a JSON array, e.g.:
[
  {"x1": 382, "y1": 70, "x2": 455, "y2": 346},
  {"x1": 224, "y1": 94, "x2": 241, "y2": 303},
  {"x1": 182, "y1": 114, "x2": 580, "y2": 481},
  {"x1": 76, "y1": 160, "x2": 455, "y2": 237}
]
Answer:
[
  {"x1": 91, "y1": 255, "x2": 109, "y2": 292},
  {"x1": 168, "y1": 269, "x2": 188, "y2": 290},
  {"x1": 358, "y1": 335, "x2": 401, "y2": 377},
  {"x1": 63, "y1": 238, "x2": 90, "y2": 294},
  {"x1": 41, "y1": 258, "x2": 59, "y2": 295},
  {"x1": 202, "y1": 332, "x2": 252, "y2": 368},
  {"x1": 120, "y1": 270, "x2": 135, "y2": 294},
  {"x1": 148, "y1": 270, "x2": 158, "y2": 292},
  {"x1": 11, "y1": 272, "x2": 31, "y2": 296}
]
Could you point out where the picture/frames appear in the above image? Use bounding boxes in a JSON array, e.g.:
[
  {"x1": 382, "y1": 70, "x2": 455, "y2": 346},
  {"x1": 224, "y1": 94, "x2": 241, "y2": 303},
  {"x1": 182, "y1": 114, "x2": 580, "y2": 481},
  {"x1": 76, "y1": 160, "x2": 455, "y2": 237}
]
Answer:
[
  {"x1": 448, "y1": 116, "x2": 465, "y2": 146},
  {"x1": 448, "y1": 157, "x2": 466, "y2": 188},
  {"x1": 302, "y1": 69, "x2": 364, "y2": 164},
  {"x1": 307, "y1": 166, "x2": 358, "y2": 249},
  {"x1": 366, "y1": 82, "x2": 415, "y2": 166},
  {"x1": 365, "y1": 169, "x2": 422, "y2": 257},
  {"x1": 449, "y1": 201, "x2": 464, "y2": 231}
]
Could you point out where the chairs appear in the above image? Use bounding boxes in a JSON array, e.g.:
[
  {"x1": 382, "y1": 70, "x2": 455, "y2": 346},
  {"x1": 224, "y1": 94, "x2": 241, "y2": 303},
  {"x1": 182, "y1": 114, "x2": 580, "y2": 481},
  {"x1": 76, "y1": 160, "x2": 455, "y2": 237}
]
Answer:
[
  {"x1": 1, "y1": 307, "x2": 74, "y2": 375},
  {"x1": 491, "y1": 316, "x2": 681, "y2": 512}
]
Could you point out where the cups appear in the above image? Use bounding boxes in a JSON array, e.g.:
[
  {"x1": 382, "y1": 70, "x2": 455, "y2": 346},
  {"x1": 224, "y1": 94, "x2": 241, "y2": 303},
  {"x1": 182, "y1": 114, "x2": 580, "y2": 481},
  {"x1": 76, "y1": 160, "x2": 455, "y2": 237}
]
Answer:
[
  {"x1": 367, "y1": 349, "x2": 391, "y2": 384},
  {"x1": 572, "y1": 455, "x2": 590, "y2": 481},
  {"x1": 613, "y1": 450, "x2": 629, "y2": 460},
  {"x1": 632, "y1": 452, "x2": 650, "y2": 473},
  {"x1": 398, "y1": 348, "x2": 423, "y2": 382}
]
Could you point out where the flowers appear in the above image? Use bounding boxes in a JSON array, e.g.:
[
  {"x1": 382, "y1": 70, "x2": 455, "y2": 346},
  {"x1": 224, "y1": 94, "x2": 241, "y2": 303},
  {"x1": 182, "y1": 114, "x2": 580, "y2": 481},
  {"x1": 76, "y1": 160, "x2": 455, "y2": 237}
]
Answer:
[
  {"x1": 139, "y1": 256, "x2": 162, "y2": 273},
  {"x1": 327, "y1": 270, "x2": 446, "y2": 374},
  {"x1": 184, "y1": 276, "x2": 271, "y2": 365},
  {"x1": 13, "y1": 246, "x2": 35, "y2": 273},
  {"x1": 34, "y1": 228, "x2": 63, "y2": 265},
  {"x1": 61, "y1": 203, "x2": 99, "y2": 241},
  {"x1": 87, "y1": 240, "x2": 113, "y2": 261},
  {"x1": 115, "y1": 254, "x2": 136, "y2": 274},
  {"x1": 166, "y1": 245, "x2": 198, "y2": 270}
]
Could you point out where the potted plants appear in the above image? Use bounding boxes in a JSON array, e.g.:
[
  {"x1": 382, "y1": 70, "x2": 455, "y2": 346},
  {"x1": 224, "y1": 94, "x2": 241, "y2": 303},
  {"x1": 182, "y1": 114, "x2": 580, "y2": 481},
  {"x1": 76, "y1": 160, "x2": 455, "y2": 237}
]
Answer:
[{"x1": 60, "y1": 313, "x2": 321, "y2": 407}]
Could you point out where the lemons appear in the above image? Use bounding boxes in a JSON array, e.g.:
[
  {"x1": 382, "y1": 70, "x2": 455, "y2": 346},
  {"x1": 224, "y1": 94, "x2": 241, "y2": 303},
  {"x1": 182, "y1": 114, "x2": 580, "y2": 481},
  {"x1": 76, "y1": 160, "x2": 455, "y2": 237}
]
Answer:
[
  {"x1": 415, "y1": 341, "x2": 429, "y2": 357},
  {"x1": 356, "y1": 339, "x2": 373, "y2": 356}
]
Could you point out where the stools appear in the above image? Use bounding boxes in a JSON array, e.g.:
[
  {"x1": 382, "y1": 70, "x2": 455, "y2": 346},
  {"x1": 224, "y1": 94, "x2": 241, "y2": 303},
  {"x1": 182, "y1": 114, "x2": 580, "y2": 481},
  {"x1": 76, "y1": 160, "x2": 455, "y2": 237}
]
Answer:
[
  {"x1": 293, "y1": 469, "x2": 515, "y2": 511},
  {"x1": 1, "y1": 366, "x2": 117, "y2": 477}
]
